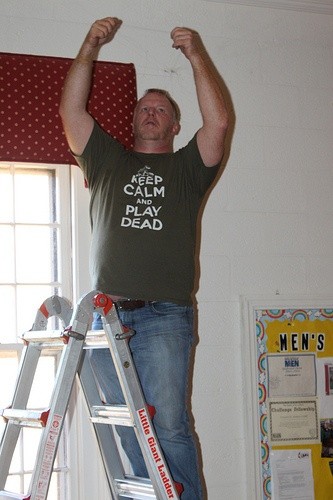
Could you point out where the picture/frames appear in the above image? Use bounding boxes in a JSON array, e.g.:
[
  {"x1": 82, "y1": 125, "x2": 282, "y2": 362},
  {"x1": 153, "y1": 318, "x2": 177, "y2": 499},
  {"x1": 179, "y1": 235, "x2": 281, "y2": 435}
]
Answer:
[{"x1": 239, "y1": 293, "x2": 332, "y2": 500}]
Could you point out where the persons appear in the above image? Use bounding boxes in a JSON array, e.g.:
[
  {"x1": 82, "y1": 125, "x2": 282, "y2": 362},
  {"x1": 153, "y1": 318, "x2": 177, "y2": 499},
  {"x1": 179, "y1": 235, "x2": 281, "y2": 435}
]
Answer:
[{"x1": 58, "y1": 16, "x2": 229, "y2": 500}]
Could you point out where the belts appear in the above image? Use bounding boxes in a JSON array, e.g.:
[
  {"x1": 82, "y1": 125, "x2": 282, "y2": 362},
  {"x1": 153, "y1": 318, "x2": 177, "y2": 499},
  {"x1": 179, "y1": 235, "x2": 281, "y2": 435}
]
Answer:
[{"x1": 112, "y1": 298, "x2": 156, "y2": 311}]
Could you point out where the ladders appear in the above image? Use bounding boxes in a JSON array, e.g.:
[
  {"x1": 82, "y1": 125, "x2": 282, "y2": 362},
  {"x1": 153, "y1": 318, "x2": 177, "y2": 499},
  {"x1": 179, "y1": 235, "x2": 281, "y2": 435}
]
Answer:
[{"x1": 0, "y1": 290, "x2": 183, "y2": 500}]
